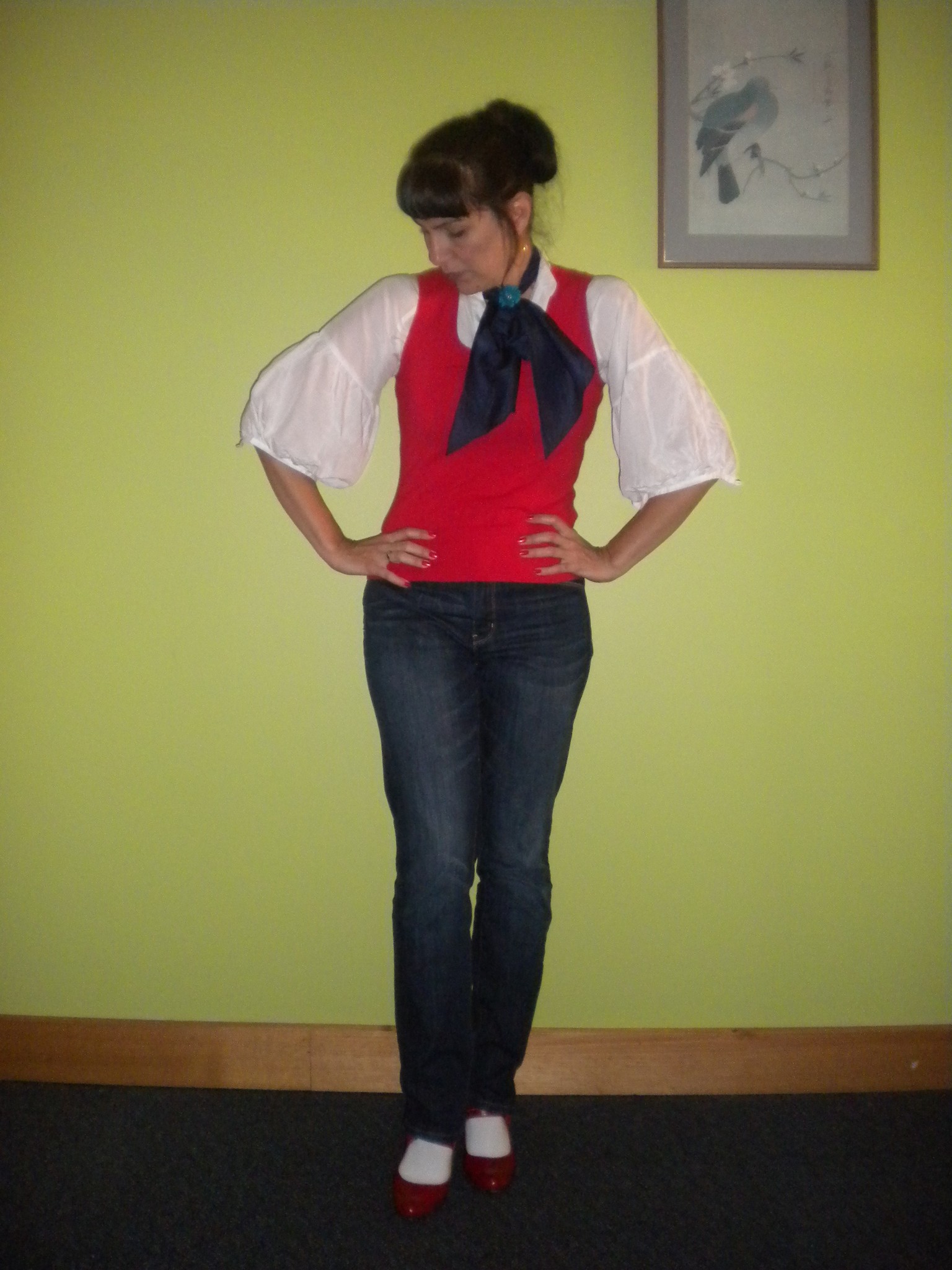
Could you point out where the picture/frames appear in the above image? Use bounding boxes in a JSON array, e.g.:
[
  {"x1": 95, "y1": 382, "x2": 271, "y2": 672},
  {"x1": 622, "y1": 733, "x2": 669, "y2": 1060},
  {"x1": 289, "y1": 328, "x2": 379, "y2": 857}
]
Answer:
[{"x1": 655, "y1": 1, "x2": 880, "y2": 270}]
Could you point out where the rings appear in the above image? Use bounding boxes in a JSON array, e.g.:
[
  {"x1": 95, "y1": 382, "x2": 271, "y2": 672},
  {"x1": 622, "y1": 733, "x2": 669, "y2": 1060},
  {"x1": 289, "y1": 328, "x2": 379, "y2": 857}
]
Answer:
[{"x1": 387, "y1": 550, "x2": 393, "y2": 563}]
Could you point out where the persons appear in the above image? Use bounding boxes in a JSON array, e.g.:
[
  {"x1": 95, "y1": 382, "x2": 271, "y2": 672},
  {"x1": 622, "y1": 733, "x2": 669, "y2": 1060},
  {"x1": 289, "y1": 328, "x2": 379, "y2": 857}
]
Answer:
[{"x1": 236, "y1": 96, "x2": 744, "y2": 1217}]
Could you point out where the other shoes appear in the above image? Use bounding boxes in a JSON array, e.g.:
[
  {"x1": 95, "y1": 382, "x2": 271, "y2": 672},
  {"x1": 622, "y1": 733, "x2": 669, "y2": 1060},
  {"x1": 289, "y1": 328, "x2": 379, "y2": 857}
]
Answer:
[
  {"x1": 394, "y1": 1133, "x2": 455, "y2": 1216},
  {"x1": 462, "y1": 1106, "x2": 516, "y2": 1192}
]
